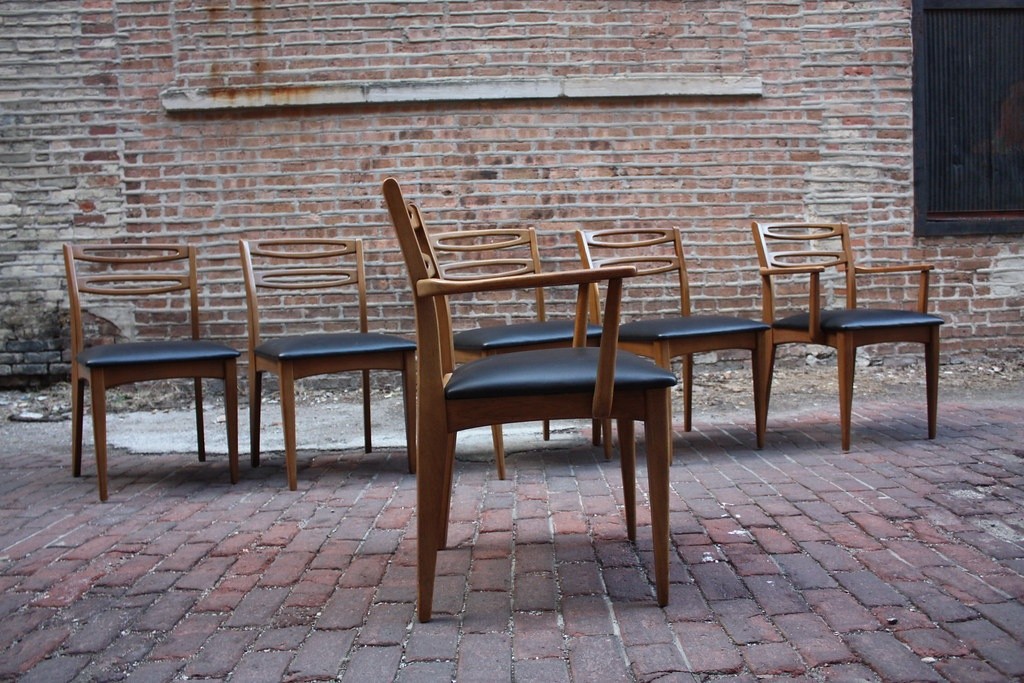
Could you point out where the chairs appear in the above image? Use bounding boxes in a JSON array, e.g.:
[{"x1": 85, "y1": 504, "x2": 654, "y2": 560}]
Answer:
[
  {"x1": 62, "y1": 242, "x2": 240, "y2": 504},
  {"x1": 239, "y1": 238, "x2": 417, "y2": 490},
  {"x1": 578, "y1": 225, "x2": 771, "y2": 466},
  {"x1": 428, "y1": 227, "x2": 611, "y2": 483},
  {"x1": 752, "y1": 221, "x2": 944, "y2": 452},
  {"x1": 384, "y1": 176, "x2": 678, "y2": 625}
]
